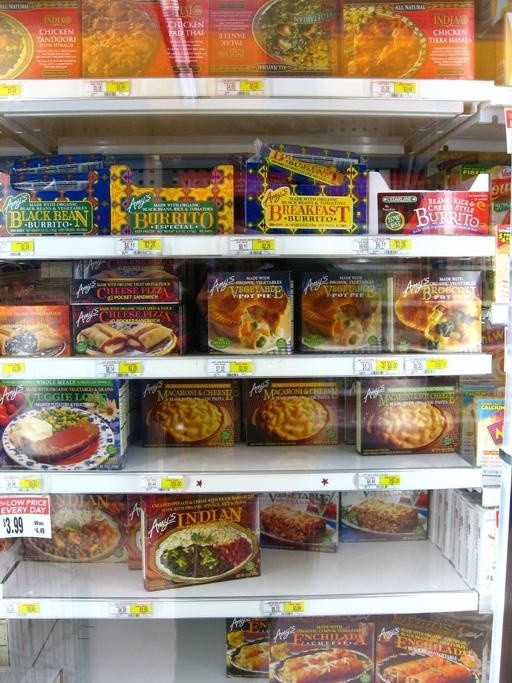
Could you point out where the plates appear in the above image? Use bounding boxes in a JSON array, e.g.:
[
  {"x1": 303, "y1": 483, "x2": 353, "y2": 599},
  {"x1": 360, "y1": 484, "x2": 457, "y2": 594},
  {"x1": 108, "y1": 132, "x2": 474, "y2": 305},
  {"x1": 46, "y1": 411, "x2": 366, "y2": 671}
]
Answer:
[
  {"x1": 0, "y1": 322, "x2": 174, "y2": 357},
  {"x1": 0, "y1": 11, "x2": 34, "y2": 80},
  {"x1": 129, "y1": 9, "x2": 157, "y2": 79},
  {"x1": 344, "y1": 6, "x2": 427, "y2": 79},
  {"x1": 1, "y1": 406, "x2": 116, "y2": 472},
  {"x1": 253, "y1": 2, "x2": 331, "y2": 71}
]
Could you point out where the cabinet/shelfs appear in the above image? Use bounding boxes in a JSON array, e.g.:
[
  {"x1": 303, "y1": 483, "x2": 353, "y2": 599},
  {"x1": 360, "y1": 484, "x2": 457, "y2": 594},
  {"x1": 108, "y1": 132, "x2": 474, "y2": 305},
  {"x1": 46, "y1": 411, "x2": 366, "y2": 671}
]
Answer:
[{"x1": 0, "y1": 78, "x2": 511, "y2": 619}]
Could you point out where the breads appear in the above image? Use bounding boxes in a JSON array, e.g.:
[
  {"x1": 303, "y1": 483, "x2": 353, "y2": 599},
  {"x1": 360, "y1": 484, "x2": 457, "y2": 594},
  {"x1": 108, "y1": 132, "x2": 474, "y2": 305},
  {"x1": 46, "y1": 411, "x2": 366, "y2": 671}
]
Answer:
[
  {"x1": 353, "y1": 499, "x2": 418, "y2": 534},
  {"x1": 82, "y1": 323, "x2": 127, "y2": 354},
  {"x1": 125, "y1": 321, "x2": 172, "y2": 352},
  {"x1": 260, "y1": 506, "x2": 327, "y2": 543}
]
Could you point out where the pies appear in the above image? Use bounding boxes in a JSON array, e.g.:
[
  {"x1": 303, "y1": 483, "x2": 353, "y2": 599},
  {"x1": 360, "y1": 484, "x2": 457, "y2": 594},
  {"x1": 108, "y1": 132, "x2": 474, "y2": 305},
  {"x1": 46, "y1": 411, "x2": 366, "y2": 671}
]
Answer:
[{"x1": 226, "y1": 631, "x2": 473, "y2": 683}]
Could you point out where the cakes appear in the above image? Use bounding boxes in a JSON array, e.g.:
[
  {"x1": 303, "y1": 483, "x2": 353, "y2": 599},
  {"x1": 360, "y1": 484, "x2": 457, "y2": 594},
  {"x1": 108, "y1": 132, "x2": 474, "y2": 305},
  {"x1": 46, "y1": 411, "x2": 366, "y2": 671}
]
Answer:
[
  {"x1": 300, "y1": 284, "x2": 380, "y2": 346},
  {"x1": 208, "y1": 283, "x2": 288, "y2": 349},
  {"x1": 395, "y1": 283, "x2": 484, "y2": 350}
]
